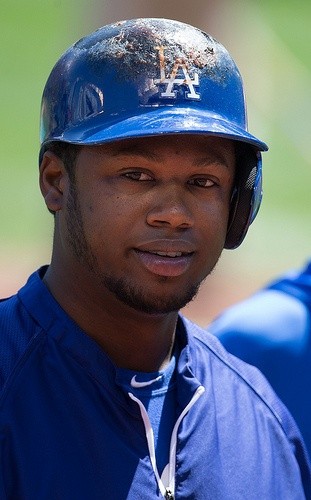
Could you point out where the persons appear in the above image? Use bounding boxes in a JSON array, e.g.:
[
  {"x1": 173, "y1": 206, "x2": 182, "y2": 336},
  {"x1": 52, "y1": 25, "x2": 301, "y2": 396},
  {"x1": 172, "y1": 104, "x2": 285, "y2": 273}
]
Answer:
[
  {"x1": 0, "y1": 15, "x2": 311, "y2": 499},
  {"x1": 210, "y1": 262, "x2": 308, "y2": 460}
]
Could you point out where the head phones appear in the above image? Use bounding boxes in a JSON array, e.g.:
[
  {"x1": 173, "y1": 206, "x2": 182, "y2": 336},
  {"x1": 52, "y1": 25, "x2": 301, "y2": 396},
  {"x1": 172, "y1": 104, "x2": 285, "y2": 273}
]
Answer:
[{"x1": 222, "y1": 140, "x2": 264, "y2": 251}]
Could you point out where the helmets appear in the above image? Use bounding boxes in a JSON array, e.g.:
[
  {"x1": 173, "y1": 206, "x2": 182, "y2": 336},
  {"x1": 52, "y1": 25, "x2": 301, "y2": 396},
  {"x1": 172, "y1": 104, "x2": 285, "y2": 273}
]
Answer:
[{"x1": 37, "y1": 19, "x2": 267, "y2": 164}]
